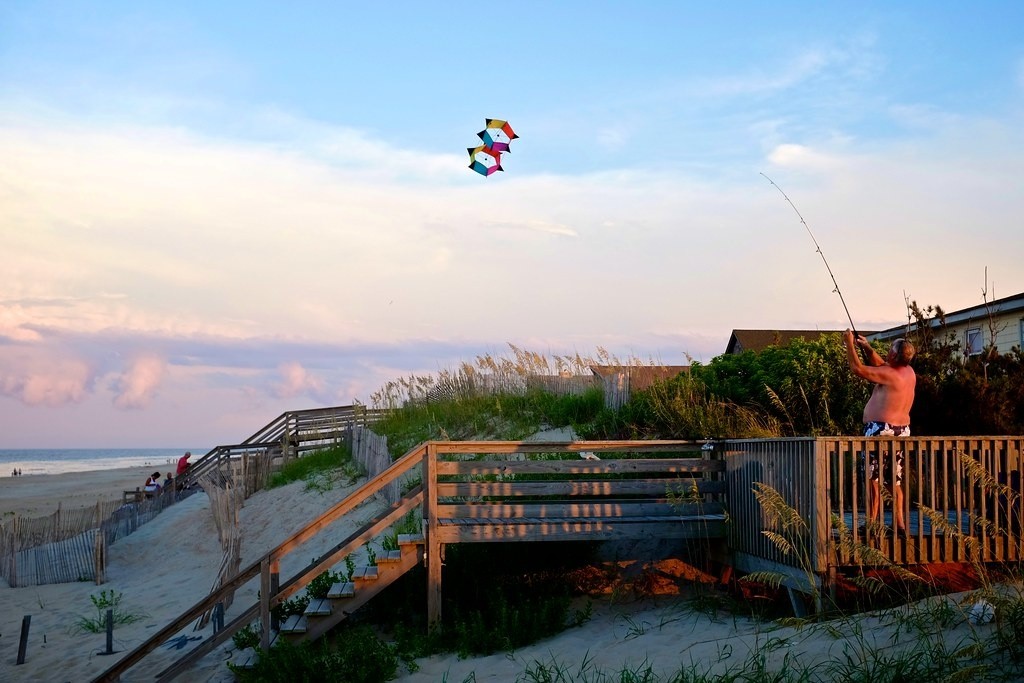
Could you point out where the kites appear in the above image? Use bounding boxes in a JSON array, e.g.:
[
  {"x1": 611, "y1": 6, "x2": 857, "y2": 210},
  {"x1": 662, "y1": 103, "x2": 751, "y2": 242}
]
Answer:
[{"x1": 467, "y1": 119, "x2": 519, "y2": 176}]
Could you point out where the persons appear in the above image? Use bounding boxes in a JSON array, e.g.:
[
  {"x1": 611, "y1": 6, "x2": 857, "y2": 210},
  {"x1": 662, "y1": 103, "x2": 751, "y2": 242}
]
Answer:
[
  {"x1": 842, "y1": 328, "x2": 916, "y2": 536},
  {"x1": 177, "y1": 452, "x2": 197, "y2": 491},
  {"x1": 146, "y1": 471, "x2": 162, "y2": 499},
  {"x1": 164, "y1": 472, "x2": 173, "y2": 486}
]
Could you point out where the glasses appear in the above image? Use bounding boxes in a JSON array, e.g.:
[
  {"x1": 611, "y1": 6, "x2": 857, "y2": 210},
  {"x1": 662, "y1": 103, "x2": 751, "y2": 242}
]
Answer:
[{"x1": 894, "y1": 339, "x2": 912, "y2": 359}]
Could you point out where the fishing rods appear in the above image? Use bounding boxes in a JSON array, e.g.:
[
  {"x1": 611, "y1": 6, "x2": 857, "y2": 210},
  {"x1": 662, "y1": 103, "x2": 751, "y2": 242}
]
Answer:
[{"x1": 755, "y1": 169, "x2": 871, "y2": 366}]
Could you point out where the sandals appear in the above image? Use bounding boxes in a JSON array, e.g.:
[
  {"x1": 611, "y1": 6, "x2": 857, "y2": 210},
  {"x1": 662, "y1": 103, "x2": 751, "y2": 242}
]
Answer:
[
  {"x1": 885, "y1": 524, "x2": 906, "y2": 535},
  {"x1": 851, "y1": 524, "x2": 875, "y2": 536}
]
